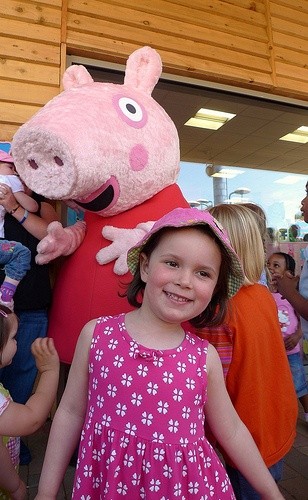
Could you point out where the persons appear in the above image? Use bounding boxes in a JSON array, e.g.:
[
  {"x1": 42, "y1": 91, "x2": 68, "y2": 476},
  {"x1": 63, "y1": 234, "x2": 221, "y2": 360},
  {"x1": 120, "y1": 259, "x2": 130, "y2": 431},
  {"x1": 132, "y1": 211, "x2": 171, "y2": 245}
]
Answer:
[
  {"x1": 34, "y1": 206, "x2": 286, "y2": 500},
  {"x1": 0, "y1": 140, "x2": 68, "y2": 500},
  {"x1": 194, "y1": 181, "x2": 308, "y2": 500}
]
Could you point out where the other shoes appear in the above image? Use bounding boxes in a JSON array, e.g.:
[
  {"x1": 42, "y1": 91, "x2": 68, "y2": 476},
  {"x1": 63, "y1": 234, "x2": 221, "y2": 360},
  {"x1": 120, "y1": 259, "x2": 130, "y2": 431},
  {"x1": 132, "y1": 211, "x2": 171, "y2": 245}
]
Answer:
[{"x1": 0, "y1": 292, "x2": 14, "y2": 317}]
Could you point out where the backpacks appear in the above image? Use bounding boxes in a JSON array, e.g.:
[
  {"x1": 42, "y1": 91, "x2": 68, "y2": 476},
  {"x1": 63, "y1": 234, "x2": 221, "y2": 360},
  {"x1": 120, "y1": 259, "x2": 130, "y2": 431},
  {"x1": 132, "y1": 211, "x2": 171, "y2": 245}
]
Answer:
[{"x1": 271, "y1": 290, "x2": 301, "y2": 356}]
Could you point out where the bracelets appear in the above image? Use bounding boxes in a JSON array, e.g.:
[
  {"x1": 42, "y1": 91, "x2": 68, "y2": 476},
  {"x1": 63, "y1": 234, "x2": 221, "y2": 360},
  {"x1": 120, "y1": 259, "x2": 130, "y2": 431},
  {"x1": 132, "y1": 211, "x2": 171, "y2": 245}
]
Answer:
[
  {"x1": 10, "y1": 205, "x2": 20, "y2": 215},
  {"x1": 19, "y1": 209, "x2": 28, "y2": 224}
]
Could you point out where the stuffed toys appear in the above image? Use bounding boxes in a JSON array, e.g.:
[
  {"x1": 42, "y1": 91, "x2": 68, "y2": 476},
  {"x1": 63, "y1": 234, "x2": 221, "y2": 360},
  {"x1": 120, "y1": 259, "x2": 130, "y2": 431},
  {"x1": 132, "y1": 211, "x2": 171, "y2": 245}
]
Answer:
[{"x1": 12, "y1": 45, "x2": 191, "y2": 410}]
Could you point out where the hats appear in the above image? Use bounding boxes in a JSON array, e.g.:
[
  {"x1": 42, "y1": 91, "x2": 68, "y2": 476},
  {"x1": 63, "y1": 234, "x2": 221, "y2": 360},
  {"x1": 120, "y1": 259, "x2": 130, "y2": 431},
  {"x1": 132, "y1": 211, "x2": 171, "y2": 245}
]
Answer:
[
  {"x1": 0, "y1": 149, "x2": 14, "y2": 163},
  {"x1": 126, "y1": 207, "x2": 245, "y2": 308}
]
